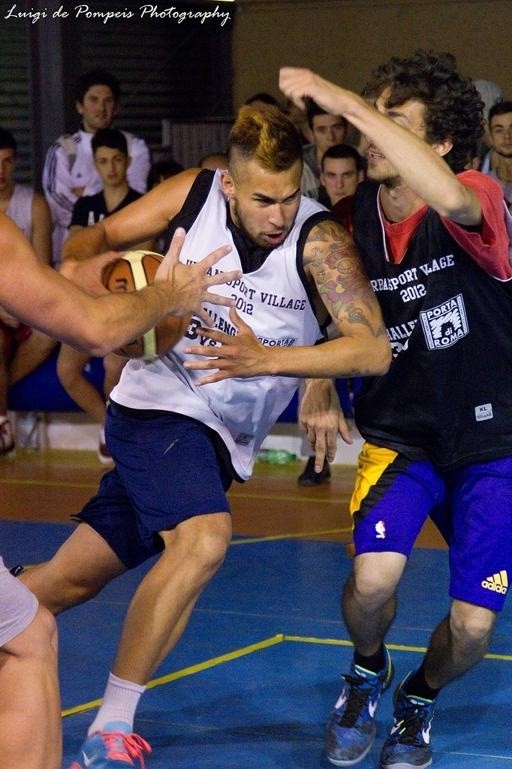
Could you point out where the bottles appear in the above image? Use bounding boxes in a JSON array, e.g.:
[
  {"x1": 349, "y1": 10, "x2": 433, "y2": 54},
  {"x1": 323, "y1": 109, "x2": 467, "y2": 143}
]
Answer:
[{"x1": 253, "y1": 450, "x2": 297, "y2": 465}]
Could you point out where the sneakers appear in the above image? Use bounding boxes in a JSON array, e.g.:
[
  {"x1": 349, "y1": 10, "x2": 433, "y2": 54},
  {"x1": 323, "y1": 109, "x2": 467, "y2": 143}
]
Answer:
[
  {"x1": 379, "y1": 671, "x2": 437, "y2": 769},
  {"x1": 297, "y1": 454, "x2": 330, "y2": 487},
  {"x1": 322, "y1": 639, "x2": 397, "y2": 768},
  {"x1": 67, "y1": 729, "x2": 139, "y2": 769}
]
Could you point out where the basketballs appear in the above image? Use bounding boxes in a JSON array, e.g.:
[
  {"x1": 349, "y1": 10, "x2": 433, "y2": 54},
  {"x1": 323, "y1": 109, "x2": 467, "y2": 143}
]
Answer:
[{"x1": 101, "y1": 250, "x2": 192, "y2": 362}]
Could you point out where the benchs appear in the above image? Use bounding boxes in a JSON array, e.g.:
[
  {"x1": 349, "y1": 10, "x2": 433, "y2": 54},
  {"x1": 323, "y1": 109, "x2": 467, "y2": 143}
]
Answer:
[{"x1": 6, "y1": 347, "x2": 364, "y2": 460}]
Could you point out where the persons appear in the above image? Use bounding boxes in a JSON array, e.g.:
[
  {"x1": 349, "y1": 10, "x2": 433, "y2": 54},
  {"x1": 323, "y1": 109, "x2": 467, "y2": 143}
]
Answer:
[
  {"x1": 0, "y1": 67, "x2": 510, "y2": 485},
  {"x1": 9, "y1": 97, "x2": 393, "y2": 767},
  {"x1": 0, "y1": 209, "x2": 247, "y2": 769},
  {"x1": 279, "y1": 46, "x2": 511, "y2": 769}
]
